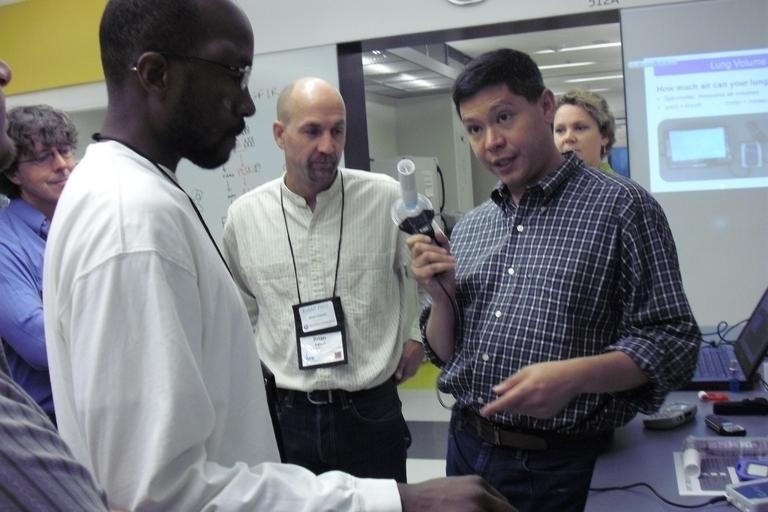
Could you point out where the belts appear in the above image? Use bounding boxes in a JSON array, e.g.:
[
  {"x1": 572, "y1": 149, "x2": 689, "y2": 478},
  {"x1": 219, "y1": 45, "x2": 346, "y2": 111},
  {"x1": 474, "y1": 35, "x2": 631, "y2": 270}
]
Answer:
[
  {"x1": 267, "y1": 384, "x2": 394, "y2": 406},
  {"x1": 462, "y1": 413, "x2": 549, "y2": 451}
]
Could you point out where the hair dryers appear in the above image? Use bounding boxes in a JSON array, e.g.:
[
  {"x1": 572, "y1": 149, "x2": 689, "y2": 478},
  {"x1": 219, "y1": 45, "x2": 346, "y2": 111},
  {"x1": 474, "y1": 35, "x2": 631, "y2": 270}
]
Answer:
[{"x1": 391, "y1": 157, "x2": 446, "y2": 277}]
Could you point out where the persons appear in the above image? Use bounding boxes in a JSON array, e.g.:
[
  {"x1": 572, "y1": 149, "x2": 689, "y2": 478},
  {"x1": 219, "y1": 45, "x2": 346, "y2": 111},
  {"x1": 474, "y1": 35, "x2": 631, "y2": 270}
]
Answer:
[
  {"x1": 0, "y1": 103, "x2": 80, "y2": 436},
  {"x1": 548, "y1": 88, "x2": 624, "y2": 177},
  {"x1": 404, "y1": 47, "x2": 704, "y2": 512},
  {"x1": 2, "y1": 57, "x2": 114, "y2": 512},
  {"x1": 40, "y1": 1, "x2": 523, "y2": 511},
  {"x1": 216, "y1": 75, "x2": 434, "y2": 488}
]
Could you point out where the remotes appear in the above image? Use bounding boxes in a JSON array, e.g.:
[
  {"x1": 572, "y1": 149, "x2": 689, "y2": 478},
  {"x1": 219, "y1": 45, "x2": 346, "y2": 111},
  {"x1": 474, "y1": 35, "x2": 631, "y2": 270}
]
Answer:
[{"x1": 703, "y1": 414, "x2": 745, "y2": 437}]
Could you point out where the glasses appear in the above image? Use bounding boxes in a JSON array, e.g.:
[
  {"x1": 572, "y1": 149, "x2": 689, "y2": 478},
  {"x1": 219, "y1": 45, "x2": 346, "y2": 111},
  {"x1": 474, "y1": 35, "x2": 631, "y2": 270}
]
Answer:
[
  {"x1": 129, "y1": 53, "x2": 252, "y2": 91},
  {"x1": 14, "y1": 144, "x2": 73, "y2": 167}
]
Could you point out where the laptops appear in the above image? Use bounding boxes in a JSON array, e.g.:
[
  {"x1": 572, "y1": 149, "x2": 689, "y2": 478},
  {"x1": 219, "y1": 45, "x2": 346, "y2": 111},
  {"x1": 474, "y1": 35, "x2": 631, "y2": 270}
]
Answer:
[{"x1": 683, "y1": 286, "x2": 768, "y2": 390}]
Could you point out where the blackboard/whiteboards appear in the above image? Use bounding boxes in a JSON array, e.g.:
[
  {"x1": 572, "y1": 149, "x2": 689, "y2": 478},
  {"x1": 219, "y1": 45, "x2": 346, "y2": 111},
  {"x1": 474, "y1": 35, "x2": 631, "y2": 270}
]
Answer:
[{"x1": 175, "y1": 45, "x2": 346, "y2": 257}]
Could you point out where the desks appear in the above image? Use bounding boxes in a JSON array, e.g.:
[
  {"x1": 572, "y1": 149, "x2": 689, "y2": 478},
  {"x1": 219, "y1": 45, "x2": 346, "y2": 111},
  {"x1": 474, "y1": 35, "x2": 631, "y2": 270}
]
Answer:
[{"x1": 585, "y1": 324, "x2": 765, "y2": 510}]
